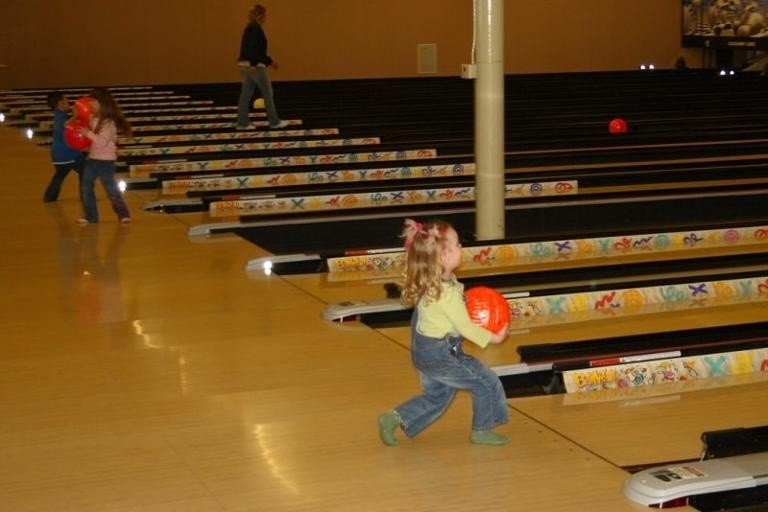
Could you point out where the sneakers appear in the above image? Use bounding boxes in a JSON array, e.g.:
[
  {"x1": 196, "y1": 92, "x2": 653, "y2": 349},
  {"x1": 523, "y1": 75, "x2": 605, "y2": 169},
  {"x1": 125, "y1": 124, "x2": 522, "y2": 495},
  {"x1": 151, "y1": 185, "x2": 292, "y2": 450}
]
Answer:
[
  {"x1": 270, "y1": 120, "x2": 289, "y2": 128},
  {"x1": 236, "y1": 125, "x2": 256, "y2": 130}
]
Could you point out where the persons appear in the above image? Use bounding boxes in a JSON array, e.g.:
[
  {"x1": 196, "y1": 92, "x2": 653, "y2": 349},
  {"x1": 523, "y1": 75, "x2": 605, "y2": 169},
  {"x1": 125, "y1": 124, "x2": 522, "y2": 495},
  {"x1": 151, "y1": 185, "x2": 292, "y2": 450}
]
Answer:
[
  {"x1": 235, "y1": 4, "x2": 290, "y2": 130},
  {"x1": 76, "y1": 85, "x2": 132, "y2": 225},
  {"x1": 41, "y1": 90, "x2": 89, "y2": 203},
  {"x1": 376, "y1": 217, "x2": 511, "y2": 447}
]
